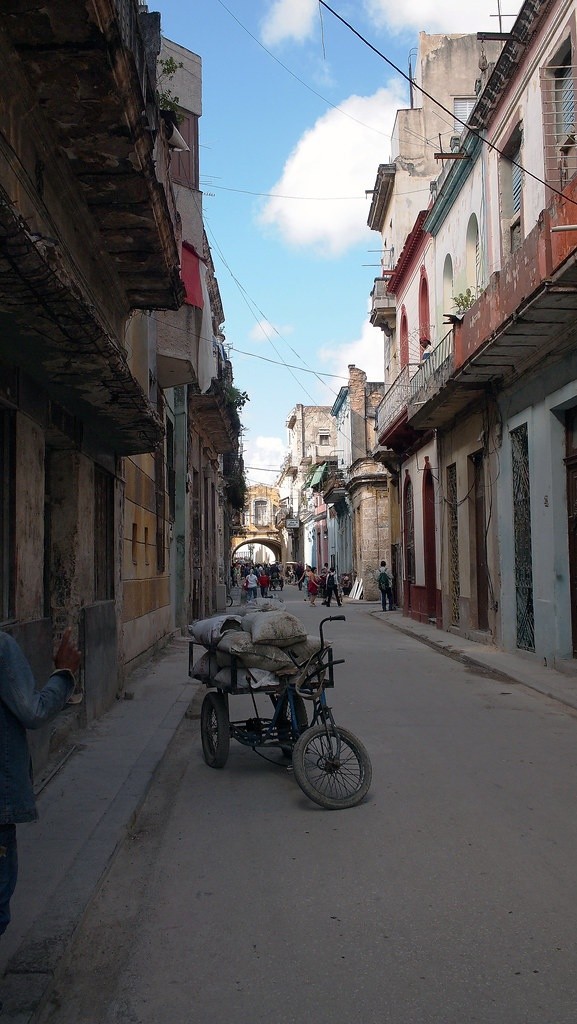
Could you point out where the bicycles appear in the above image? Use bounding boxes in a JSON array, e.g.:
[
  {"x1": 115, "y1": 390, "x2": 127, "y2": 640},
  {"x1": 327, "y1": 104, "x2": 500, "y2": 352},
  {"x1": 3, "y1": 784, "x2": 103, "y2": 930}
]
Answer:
[{"x1": 225, "y1": 582, "x2": 233, "y2": 607}]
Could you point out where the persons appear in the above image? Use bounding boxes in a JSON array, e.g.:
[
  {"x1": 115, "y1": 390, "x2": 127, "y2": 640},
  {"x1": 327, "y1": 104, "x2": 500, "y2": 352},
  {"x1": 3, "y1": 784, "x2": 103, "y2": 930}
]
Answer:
[
  {"x1": 0, "y1": 626, "x2": 82, "y2": 935},
  {"x1": 295, "y1": 562, "x2": 352, "y2": 606},
  {"x1": 231, "y1": 559, "x2": 294, "y2": 598},
  {"x1": 374, "y1": 561, "x2": 397, "y2": 611}
]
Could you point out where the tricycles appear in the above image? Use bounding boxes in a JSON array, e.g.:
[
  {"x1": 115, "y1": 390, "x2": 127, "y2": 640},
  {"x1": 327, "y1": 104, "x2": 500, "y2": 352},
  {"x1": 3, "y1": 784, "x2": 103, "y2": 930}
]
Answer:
[
  {"x1": 269, "y1": 573, "x2": 284, "y2": 591},
  {"x1": 188, "y1": 615, "x2": 372, "y2": 808}
]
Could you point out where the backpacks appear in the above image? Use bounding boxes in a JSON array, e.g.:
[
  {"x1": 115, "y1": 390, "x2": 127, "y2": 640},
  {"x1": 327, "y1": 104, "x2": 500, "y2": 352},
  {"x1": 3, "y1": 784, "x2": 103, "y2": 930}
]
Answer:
[
  {"x1": 378, "y1": 569, "x2": 389, "y2": 591},
  {"x1": 328, "y1": 574, "x2": 334, "y2": 587}
]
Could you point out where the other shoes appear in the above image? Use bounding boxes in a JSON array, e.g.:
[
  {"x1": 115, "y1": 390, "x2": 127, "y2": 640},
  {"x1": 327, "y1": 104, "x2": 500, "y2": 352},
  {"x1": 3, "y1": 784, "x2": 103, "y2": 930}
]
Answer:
[
  {"x1": 310, "y1": 603, "x2": 316, "y2": 607},
  {"x1": 322, "y1": 601, "x2": 327, "y2": 605},
  {"x1": 389, "y1": 607, "x2": 391, "y2": 610},
  {"x1": 383, "y1": 608, "x2": 386, "y2": 612}
]
